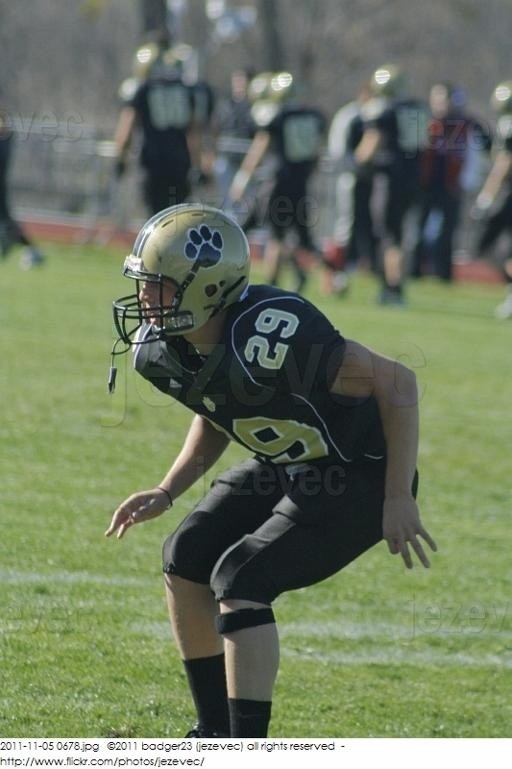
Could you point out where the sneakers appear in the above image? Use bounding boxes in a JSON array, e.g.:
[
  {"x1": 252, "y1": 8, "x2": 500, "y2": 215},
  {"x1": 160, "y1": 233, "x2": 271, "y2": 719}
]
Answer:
[
  {"x1": 17, "y1": 243, "x2": 48, "y2": 273},
  {"x1": 263, "y1": 266, "x2": 511, "y2": 320}
]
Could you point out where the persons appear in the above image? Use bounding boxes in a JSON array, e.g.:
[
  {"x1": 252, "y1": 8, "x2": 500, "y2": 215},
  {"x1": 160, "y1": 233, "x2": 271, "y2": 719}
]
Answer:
[
  {"x1": 107, "y1": 27, "x2": 219, "y2": 218},
  {"x1": 102, "y1": 199, "x2": 441, "y2": 737},
  {"x1": 1, "y1": 116, "x2": 49, "y2": 270},
  {"x1": 202, "y1": 52, "x2": 511, "y2": 319}
]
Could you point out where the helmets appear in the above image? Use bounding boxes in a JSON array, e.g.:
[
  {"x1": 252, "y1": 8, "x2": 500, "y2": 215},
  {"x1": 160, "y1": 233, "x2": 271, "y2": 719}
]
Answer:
[{"x1": 110, "y1": 202, "x2": 252, "y2": 345}]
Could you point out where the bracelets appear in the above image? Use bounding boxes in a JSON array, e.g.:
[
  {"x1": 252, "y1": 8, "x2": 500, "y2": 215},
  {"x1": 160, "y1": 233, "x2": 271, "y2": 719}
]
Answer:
[{"x1": 158, "y1": 487, "x2": 173, "y2": 510}]
